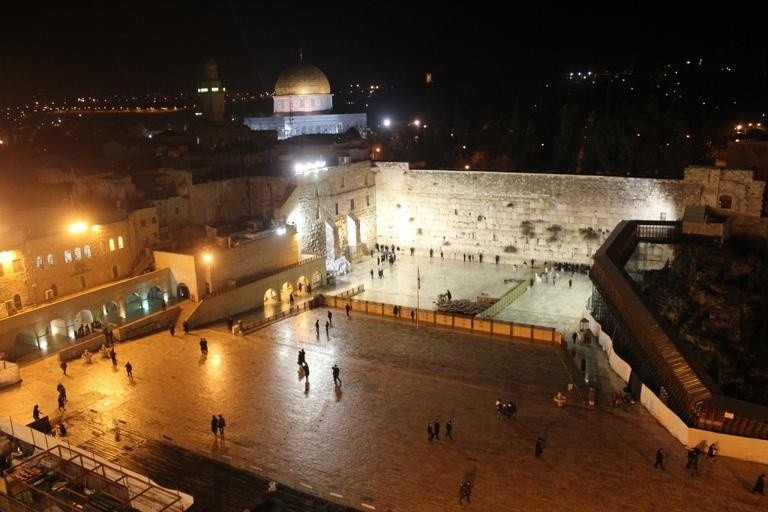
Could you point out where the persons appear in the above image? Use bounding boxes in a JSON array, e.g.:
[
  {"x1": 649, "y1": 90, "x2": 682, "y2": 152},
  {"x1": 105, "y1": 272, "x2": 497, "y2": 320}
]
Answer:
[
  {"x1": 203, "y1": 338, "x2": 208, "y2": 354},
  {"x1": 427, "y1": 422, "x2": 434, "y2": 443},
  {"x1": 57, "y1": 394, "x2": 66, "y2": 412},
  {"x1": 183, "y1": 320, "x2": 189, "y2": 336},
  {"x1": 751, "y1": 473, "x2": 768, "y2": 497},
  {"x1": 444, "y1": 420, "x2": 453, "y2": 440},
  {"x1": 33, "y1": 405, "x2": 44, "y2": 421},
  {"x1": 200, "y1": 337, "x2": 204, "y2": 352},
  {"x1": 325, "y1": 321, "x2": 329, "y2": 337},
  {"x1": 210, "y1": 414, "x2": 219, "y2": 437},
  {"x1": 169, "y1": 320, "x2": 176, "y2": 336},
  {"x1": 464, "y1": 480, "x2": 472, "y2": 503},
  {"x1": 161, "y1": 299, "x2": 167, "y2": 313},
  {"x1": 298, "y1": 283, "x2": 303, "y2": 292},
  {"x1": 653, "y1": 447, "x2": 668, "y2": 470},
  {"x1": 218, "y1": 412, "x2": 227, "y2": 436},
  {"x1": 302, "y1": 362, "x2": 309, "y2": 379},
  {"x1": 392, "y1": 305, "x2": 399, "y2": 317},
  {"x1": 495, "y1": 398, "x2": 504, "y2": 422},
  {"x1": 301, "y1": 349, "x2": 306, "y2": 364},
  {"x1": 410, "y1": 309, "x2": 414, "y2": 322},
  {"x1": 327, "y1": 310, "x2": 332, "y2": 324},
  {"x1": 109, "y1": 347, "x2": 118, "y2": 367},
  {"x1": 571, "y1": 331, "x2": 579, "y2": 344},
  {"x1": 501, "y1": 402, "x2": 508, "y2": 419},
  {"x1": 345, "y1": 303, "x2": 350, "y2": 318},
  {"x1": 332, "y1": 364, "x2": 342, "y2": 385},
  {"x1": 315, "y1": 319, "x2": 320, "y2": 337},
  {"x1": 125, "y1": 361, "x2": 133, "y2": 378},
  {"x1": 60, "y1": 359, "x2": 68, "y2": 376},
  {"x1": 433, "y1": 418, "x2": 441, "y2": 441},
  {"x1": 534, "y1": 438, "x2": 543, "y2": 458},
  {"x1": 289, "y1": 293, "x2": 294, "y2": 305},
  {"x1": 298, "y1": 351, "x2": 303, "y2": 368},
  {"x1": 456, "y1": 482, "x2": 465, "y2": 507},
  {"x1": 74, "y1": 319, "x2": 113, "y2": 344},
  {"x1": 228, "y1": 316, "x2": 233, "y2": 331},
  {"x1": 56, "y1": 382, "x2": 68, "y2": 402},
  {"x1": 543, "y1": 261, "x2": 590, "y2": 289},
  {"x1": 685, "y1": 441, "x2": 718, "y2": 476},
  {"x1": 306, "y1": 283, "x2": 312, "y2": 296},
  {"x1": 368, "y1": 247, "x2": 397, "y2": 279},
  {"x1": 506, "y1": 404, "x2": 515, "y2": 420}
]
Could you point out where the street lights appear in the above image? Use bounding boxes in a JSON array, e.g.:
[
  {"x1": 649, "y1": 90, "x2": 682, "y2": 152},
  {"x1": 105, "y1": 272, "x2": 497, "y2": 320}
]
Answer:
[{"x1": 202, "y1": 247, "x2": 213, "y2": 295}]
